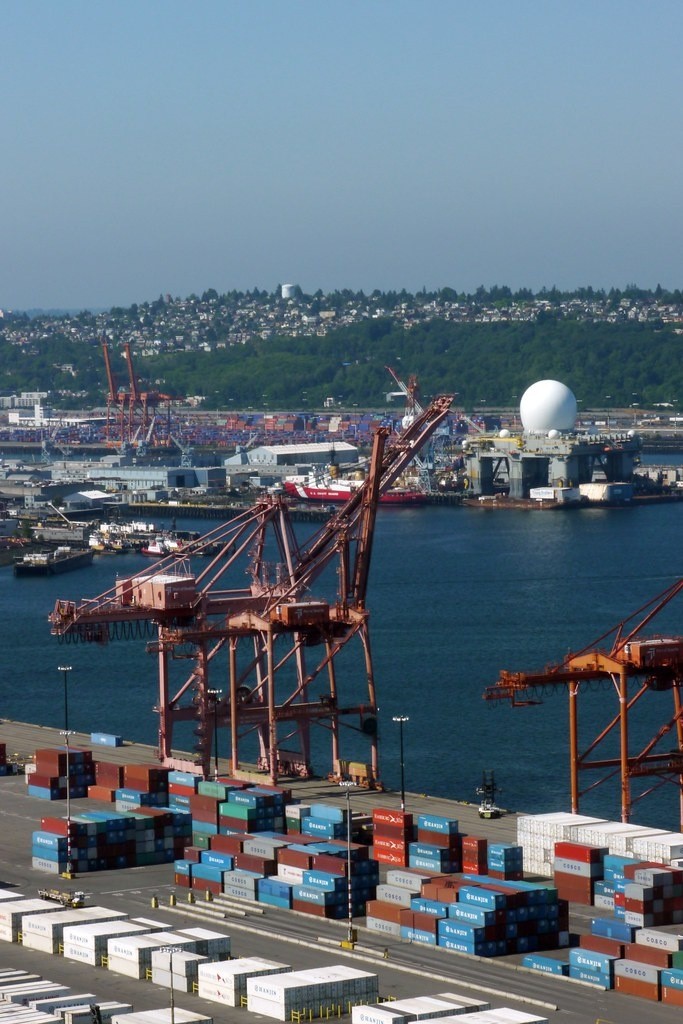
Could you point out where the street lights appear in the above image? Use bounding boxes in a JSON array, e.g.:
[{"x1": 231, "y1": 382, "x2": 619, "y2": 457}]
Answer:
[
  {"x1": 57, "y1": 664, "x2": 74, "y2": 875},
  {"x1": 338, "y1": 778, "x2": 358, "y2": 942},
  {"x1": 207, "y1": 686, "x2": 223, "y2": 781},
  {"x1": 392, "y1": 713, "x2": 411, "y2": 811}
]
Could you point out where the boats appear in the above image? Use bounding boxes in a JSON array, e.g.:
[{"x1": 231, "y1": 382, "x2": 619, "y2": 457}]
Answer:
[
  {"x1": 13, "y1": 546, "x2": 97, "y2": 578},
  {"x1": 0, "y1": 457, "x2": 472, "y2": 565}
]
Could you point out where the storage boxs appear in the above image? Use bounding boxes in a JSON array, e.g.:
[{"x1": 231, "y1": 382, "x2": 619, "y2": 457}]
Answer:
[
  {"x1": 114, "y1": 573, "x2": 196, "y2": 609},
  {"x1": 1, "y1": 411, "x2": 504, "y2": 450},
  {"x1": 0, "y1": 731, "x2": 683, "y2": 1024}
]
